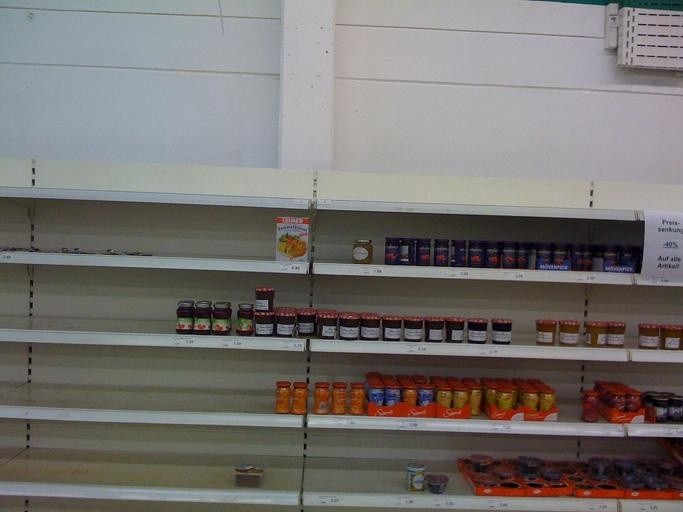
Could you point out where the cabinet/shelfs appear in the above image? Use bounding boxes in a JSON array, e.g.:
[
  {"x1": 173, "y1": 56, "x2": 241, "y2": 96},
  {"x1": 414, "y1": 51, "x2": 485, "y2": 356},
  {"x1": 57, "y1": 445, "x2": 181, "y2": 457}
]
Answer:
[{"x1": 0, "y1": 157, "x2": 683, "y2": 511}]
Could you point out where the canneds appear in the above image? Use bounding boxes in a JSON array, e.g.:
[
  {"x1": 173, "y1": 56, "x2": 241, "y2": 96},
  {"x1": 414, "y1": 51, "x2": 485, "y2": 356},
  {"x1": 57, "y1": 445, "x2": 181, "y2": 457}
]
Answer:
[
  {"x1": 276, "y1": 308, "x2": 512, "y2": 344},
  {"x1": 535, "y1": 319, "x2": 580, "y2": 347},
  {"x1": 275, "y1": 382, "x2": 307, "y2": 414},
  {"x1": 581, "y1": 380, "x2": 641, "y2": 423},
  {"x1": 176, "y1": 301, "x2": 232, "y2": 335},
  {"x1": 638, "y1": 323, "x2": 683, "y2": 349},
  {"x1": 406, "y1": 463, "x2": 426, "y2": 491},
  {"x1": 352, "y1": 239, "x2": 373, "y2": 263},
  {"x1": 312, "y1": 381, "x2": 365, "y2": 414},
  {"x1": 584, "y1": 322, "x2": 625, "y2": 347},
  {"x1": 236, "y1": 287, "x2": 276, "y2": 338},
  {"x1": 365, "y1": 372, "x2": 555, "y2": 415},
  {"x1": 645, "y1": 391, "x2": 683, "y2": 422},
  {"x1": 385, "y1": 237, "x2": 641, "y2": 272}
]
